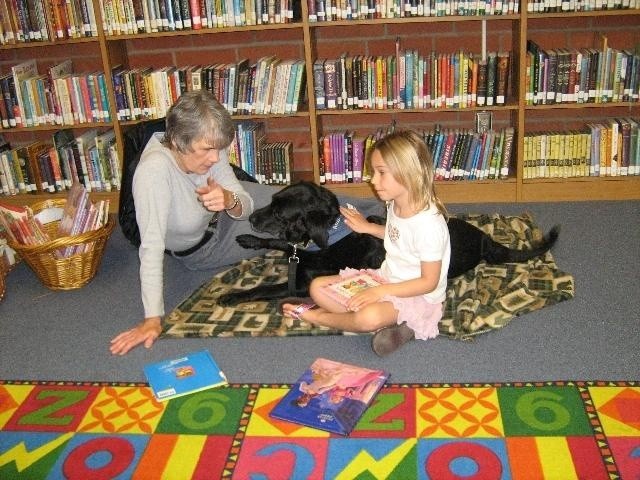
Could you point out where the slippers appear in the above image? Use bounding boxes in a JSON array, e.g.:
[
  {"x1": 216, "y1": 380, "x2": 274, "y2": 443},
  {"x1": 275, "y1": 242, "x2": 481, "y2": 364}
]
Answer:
[
  {"x1": 372, "y1": 321, "x2": 415, "y2": 358},
  {"x1": 278, "y1": 297, "x2": 320, "y2": 322}
]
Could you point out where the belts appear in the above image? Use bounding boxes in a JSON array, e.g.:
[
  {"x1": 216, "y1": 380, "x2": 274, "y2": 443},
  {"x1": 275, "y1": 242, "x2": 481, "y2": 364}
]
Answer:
[{"x1": 164, "y1": 211, "x2": 218, "y2": 256}]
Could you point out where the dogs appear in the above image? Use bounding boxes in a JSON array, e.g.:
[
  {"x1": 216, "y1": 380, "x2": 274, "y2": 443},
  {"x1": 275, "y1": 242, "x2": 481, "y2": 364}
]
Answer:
[{"x1": 216, "y1": 180, "x2": 560, "y2": 307}]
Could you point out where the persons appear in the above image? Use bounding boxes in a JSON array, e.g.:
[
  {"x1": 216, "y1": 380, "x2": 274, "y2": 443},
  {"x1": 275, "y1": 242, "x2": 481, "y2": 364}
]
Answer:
[
  {"x1": 107, "y1": 90, "x2": 391, "y2": 356},
  {"x1": 280, "y1": 130, "x2": 453, "y2": 355}
]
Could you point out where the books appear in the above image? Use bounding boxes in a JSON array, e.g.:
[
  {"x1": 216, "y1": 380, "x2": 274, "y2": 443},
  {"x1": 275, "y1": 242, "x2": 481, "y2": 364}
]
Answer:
[
  {"x1": 524, "y1": 1, "x2": 640, "y2": 178},
  {"x1": 1, "y1": 129, "x2": 121, "y2": 197},
  {"x1": 142, "y1": 347, "x2": 229, "y2": 402},
  {"x1": 320, "y1": 268, "x2": 388, "y2": 307},
  {"x1": 98, "y1": 0, "x2": 305, "y2": 187},
  {"x1": 1, "y1": 184, "x2": 109, "y2": 258},
  {"x1": 0, "y1": 0, "x2": 111, "y2": 128},
  {"x1": 270, "y1": 356, "x2": 392, "y2": 437},
  {"x1": 309, "y1": 0, "x2": 520, "y2": 184}
]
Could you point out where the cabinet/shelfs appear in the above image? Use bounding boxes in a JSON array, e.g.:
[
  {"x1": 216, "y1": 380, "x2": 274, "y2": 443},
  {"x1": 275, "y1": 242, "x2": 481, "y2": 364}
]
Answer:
[{"x1": 0, "y1": 0, "x2": 640, "y2": 214}]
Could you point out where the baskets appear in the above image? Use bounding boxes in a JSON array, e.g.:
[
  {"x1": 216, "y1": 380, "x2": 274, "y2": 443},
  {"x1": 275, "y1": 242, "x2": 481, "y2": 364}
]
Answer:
[{"x1": 6, "y1": 198, "x2": 117, "y2": 290}]
